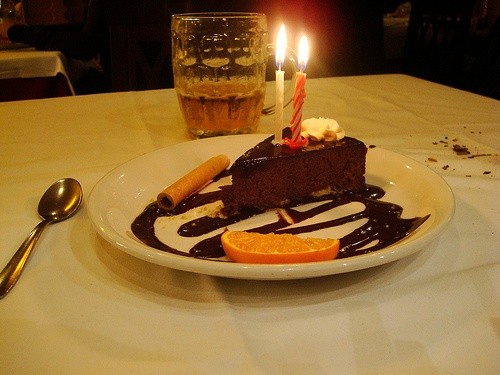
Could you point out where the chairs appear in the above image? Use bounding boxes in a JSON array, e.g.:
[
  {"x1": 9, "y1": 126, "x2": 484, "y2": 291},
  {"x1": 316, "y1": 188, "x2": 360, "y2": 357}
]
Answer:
[
  {"x1": 352, "y1": 0, "x2": 499, "y2": 100},
  {"x1": 109, "y1": 22, "x2": 174, "y2": 91}
]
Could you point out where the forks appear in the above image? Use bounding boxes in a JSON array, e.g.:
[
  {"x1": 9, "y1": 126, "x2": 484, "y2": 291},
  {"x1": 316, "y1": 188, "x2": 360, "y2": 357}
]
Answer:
[{"x1": 262, "y1": 99, "x2": 293, "y2": 115}]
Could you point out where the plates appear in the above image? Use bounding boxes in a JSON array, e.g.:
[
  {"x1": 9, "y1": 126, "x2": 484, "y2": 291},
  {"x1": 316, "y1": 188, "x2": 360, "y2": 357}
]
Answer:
[{"x1": 85, "y1": 133, "x2": 456, "y2": 280}]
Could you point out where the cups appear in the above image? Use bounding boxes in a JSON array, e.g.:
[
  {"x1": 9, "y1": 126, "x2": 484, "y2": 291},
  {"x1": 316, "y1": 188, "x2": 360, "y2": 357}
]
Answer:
[{"x1": 171, "y1": 12, "x2": 268, "y2": 141}]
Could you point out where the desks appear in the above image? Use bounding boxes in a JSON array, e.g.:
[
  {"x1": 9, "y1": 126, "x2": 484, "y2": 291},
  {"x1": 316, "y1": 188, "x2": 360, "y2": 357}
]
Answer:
[
  {"x1": 383, "y1": 14, "x2": 481, "y2": 60},
  {"x1": 0, "y1": 50, "x2": 76, "y2": 97}
]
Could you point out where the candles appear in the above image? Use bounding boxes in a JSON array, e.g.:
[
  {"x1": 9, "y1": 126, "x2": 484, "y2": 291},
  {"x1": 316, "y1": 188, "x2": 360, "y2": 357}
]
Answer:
[
  {"x1": 274, "y1": 25, "x2": 287, "y2": 142},
  {"x1": 291, "y1": 35, "x2": 310, "y2": 143}
]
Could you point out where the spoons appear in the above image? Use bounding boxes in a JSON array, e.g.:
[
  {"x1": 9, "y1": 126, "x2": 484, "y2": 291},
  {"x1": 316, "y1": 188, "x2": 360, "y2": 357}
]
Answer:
[{"x1": 0, "y1": 178, "x2": 83, "y2": 299}]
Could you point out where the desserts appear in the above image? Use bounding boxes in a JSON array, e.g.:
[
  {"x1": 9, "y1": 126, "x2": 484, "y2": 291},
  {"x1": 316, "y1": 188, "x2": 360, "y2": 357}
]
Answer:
[{"x1": 219, "y1": 117, "x2": 368, "y2": 216}]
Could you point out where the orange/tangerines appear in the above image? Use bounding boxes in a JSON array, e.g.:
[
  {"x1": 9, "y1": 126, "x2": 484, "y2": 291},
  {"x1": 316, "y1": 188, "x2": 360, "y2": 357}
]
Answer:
[{"x1": 221, "y1": 231, "x2": 339, "y2": 264}]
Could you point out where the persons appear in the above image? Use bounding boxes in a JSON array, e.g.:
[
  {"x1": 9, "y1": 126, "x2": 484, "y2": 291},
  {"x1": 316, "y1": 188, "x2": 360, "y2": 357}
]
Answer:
[{"x1": 0, "y1": 0, "x2": 151, "y2": 96}]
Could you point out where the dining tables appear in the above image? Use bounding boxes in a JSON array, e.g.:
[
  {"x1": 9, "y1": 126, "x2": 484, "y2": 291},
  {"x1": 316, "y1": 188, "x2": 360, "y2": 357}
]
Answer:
[{"x1": 0, "y1": 74, "x2": 500, "y2": 375}]
